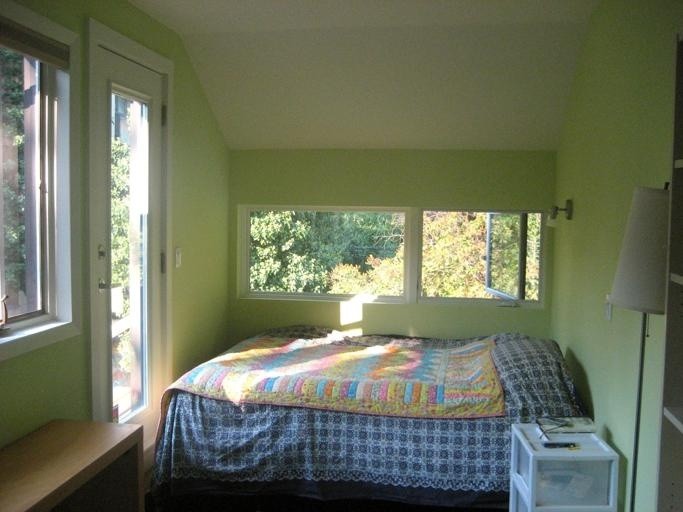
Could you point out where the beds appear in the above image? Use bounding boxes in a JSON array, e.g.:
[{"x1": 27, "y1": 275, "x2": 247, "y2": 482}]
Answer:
[{"x1": 150, "y1": 325, "x2": 586, "y2": 506}]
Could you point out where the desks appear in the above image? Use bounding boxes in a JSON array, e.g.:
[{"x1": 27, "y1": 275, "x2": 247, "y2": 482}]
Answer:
[{"x1": 0, "y1": 419, "x2": 145, "y2": 512}]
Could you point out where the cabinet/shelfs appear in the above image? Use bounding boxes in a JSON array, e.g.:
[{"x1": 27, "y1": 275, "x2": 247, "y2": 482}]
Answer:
[{"x1": 509, "y1": 423, "x2": 618, "y2": 512}]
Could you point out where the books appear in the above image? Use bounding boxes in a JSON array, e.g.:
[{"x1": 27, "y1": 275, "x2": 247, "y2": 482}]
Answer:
[{"x1": 536, "y1": 416, "x2": 597, "y2": 433}]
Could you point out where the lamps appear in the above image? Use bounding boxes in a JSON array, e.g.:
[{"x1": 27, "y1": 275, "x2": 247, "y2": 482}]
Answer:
[
  {"x1": 607, "y1": 180, "x2": 669, "y2": 507},
  {"x1": 546, "y1": 199, "x2": 573, "y2": 228}
]
